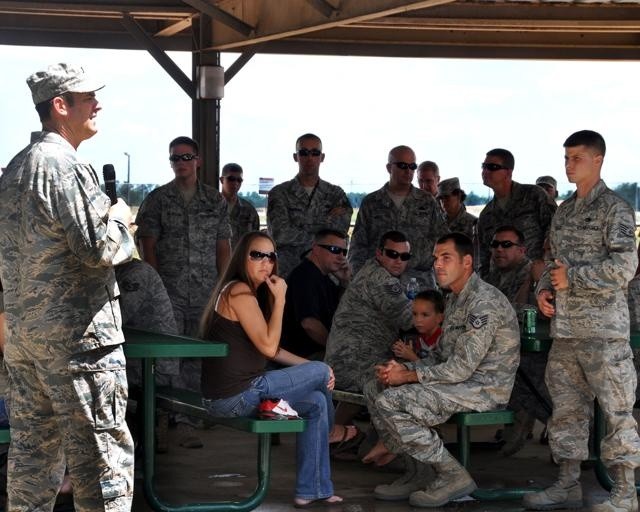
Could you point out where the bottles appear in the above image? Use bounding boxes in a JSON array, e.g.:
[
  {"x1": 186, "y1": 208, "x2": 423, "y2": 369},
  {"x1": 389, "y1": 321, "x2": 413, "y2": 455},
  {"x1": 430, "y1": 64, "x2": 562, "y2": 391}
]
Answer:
[
  {"x1": 522, "y1": 309, "x2": 536, "y2": 336},
  {"x1": 407, "y1": 278, "x2": 419, "y2": 299}
]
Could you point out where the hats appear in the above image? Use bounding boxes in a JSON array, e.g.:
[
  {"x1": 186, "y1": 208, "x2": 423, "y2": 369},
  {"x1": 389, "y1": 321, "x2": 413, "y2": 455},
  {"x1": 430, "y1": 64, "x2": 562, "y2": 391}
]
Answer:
[
  {"x1": 535, "y1": 175, "x2": 557, "y2": 188},
  {"x1": 436, "y1": 177, "x2": 460, "y2": 196},
  {"x1": 26, "y1": 62, "x2": 107, "y2": 105}
]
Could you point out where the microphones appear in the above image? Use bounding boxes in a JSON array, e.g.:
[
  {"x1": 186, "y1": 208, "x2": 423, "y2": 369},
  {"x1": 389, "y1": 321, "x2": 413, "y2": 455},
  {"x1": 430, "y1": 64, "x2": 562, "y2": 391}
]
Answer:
[{"x1": 102, "y1": 164, "x2": 118, "y2": 205}]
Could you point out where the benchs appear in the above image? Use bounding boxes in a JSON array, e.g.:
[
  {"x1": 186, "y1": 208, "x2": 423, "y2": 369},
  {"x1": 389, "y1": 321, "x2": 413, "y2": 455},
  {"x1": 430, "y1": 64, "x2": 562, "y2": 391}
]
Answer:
[
  {"x1": 328, "y1": 388, "x2": 515, "y2": 503},
  {"x1": 131, "y1": 385, "x2": 307, "y2": 512}
]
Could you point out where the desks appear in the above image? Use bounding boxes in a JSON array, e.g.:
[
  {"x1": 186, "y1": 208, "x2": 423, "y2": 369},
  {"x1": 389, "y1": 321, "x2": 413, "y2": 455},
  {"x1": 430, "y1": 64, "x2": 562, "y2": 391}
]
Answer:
[
  {"x1": 122, "y1": 332, "x2": 227, "y2": 511},
  {"x1": 515, "y1": 327, "x2": 640, "y2": 502}
]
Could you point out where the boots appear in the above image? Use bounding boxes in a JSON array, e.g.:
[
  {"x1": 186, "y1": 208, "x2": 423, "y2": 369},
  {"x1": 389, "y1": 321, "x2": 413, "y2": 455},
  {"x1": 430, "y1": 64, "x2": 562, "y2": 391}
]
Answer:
[
  {"x1": 407, "y1": 453, "x2": 477, "y2": 506},
  {"x1": 592, "y1": 463, "x2": 639, "y2": 512},
  {"x1": 522, "y1": 464, "x2": 584, "y2": 509},
  {"x1": 376, "y1": 453, "x2": 426, "y2": 500}
]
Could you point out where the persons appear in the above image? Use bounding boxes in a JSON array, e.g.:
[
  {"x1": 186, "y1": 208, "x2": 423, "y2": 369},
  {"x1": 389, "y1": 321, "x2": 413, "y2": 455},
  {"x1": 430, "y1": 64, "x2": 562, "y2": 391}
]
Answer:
[
  {"x1": 525, "y1": 129, "x2": 639, "y2": 512},
  {"x1": 134, "y1": 136, "x2": 233, "y2": 453},
  {"x1": 475, "y1": 147, "x2": 558, "y2": 259},
  {"x1": 417, "y1": 159, "x2": 441, "y2": 197},
  {"x1": 268, "y1": 134, "x2": 353, "y2": 274},
  {"x1": 437, "y1": 177, "x2": 479, "y2": 239},
  {"x1": 200, "y1": 229, "x2": 360, "y2": 508},
  {"x1": 482, "y1": 228, "x2": 538, "y2": 337},
  {"x1": 537, "y1": 176, "x2": 559, "y2": 203},
  {"x1": 372, "y1": 233, "x2": 521, "y2": 509},
  {"x1": 219, "y1": 162, "x2": 261, "y2": 241},
  {"x1": 324, "y1": 230, "x2": 415, "y2": 396},
  {"x1": 364, "y1": 288, "x2": 446, "y2": 468},
  {"x1": 0, "y1": 60, "x2": 138, "y2": 512},
  {"x1": 112, "y1": 240, "x2": 179, "y2": 391},
  {"x1": 284, "y1": 226, "x2": 353, "y2": 369},
  {"x1": 350, "y1": 145, "x2": 449, "y2": 298}
]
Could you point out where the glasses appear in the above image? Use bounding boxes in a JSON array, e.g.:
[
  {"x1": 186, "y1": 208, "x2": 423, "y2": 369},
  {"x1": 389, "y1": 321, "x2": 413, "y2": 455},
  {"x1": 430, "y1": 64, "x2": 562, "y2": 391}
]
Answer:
[
  {"x1": 317, "y1": 244, "x2": 348, "y2": 256},
  {"x1": 299, "y1": 148, "x2": 322, "y2": 156},
  {"x1": 228, "y1": 176, "x2": 243, "y2": 182},
  {"x1": 382, "y1": 247, "x2": 410, "y2": 261},
  {"x1": 169, "y1": 153, "x2": 195, "y2": 161},
  {"x1": 491, "y1": 239, "x2": 519, "y2": 248},
  {"x1": 250, "y1": 250, "x2": 276, "y2": 262},
  {"x1": 393, "y1": 162, "x2": 418, "y2": 170},
  {"x1": 482, "y1": 163, "x2": 507, "y2": 171}
]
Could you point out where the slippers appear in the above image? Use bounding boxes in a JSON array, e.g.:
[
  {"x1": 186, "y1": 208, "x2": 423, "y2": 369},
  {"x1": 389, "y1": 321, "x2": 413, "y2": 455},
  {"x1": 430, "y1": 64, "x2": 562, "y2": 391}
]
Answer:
[
  {"x1": 330, "y1": 422, "x2": 359, "y2": 446},
  {"x1": 295, "y1": 493, "x2": 344, "y2": 508}
]
